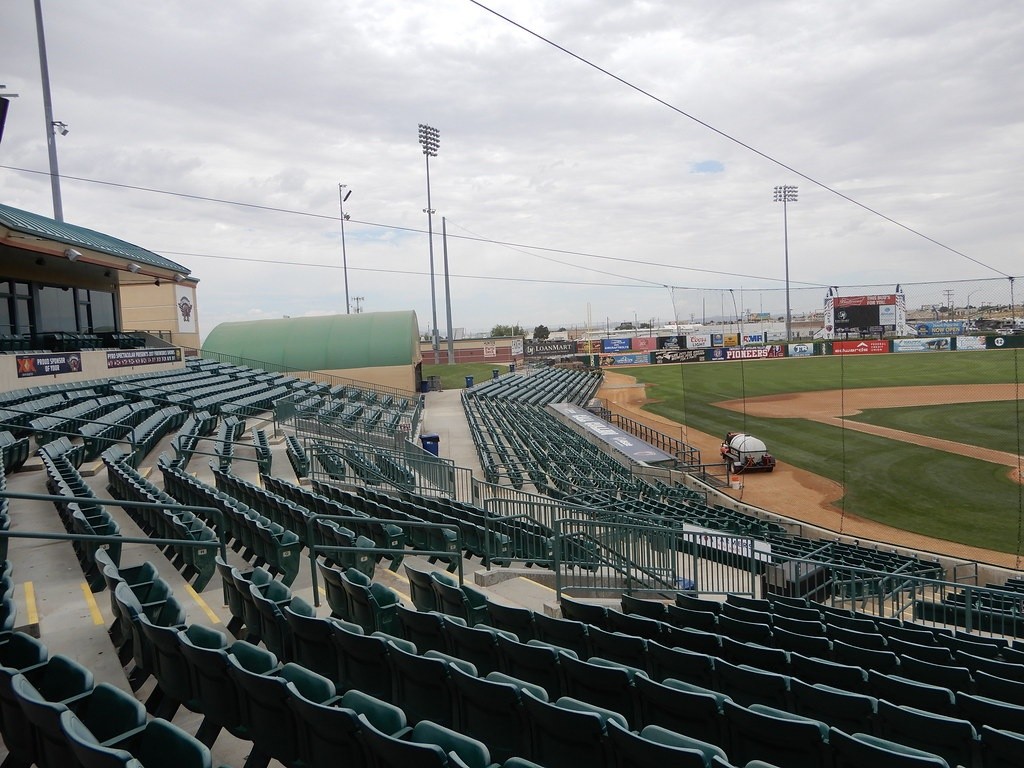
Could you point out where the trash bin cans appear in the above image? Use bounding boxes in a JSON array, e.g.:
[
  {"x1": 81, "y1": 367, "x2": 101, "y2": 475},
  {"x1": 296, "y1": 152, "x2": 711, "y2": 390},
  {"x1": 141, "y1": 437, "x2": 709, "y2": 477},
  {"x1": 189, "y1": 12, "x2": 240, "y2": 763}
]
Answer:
[
  {"x1": 677, "y1": 577, "x2": 698, "y2": 599},
  {"x1": 465, "y1": 376, "x2": 474, "y2": 389},
  {"x1": 510, "y1": 365, "x2": 514, "y2": 372},
  {"x1": 493, "y1": 369, "x2": 499, "y2": 379},
  {"x1": 421, "y1": 380, "x2": 429, "y2": 393},
  {"x1": 420, "y1": 433, "x2": 439, "y2": 462}
]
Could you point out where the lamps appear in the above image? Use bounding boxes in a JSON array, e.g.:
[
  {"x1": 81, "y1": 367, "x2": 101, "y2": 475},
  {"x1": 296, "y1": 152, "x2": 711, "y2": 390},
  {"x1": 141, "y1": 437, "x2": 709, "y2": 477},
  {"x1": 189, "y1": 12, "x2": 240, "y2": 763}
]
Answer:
[
  {"x1": 173, "y1": 273, "x2": 186, "y2": 284},
  {"x1": 64, "y1": 249, "x2": 83, "y2": 263},
  {"x1": 127, "y1": 263, "x2": 142, "y2": 274},
  {"x1": 103, "y1": 271, "x2": 113, "y2": 278},
  {"x1": 37, "y1": 257, "x2": 45, "y2": 265},
  {"x1": 154, "y1": 277, "x2": 160, "y2": 287}
]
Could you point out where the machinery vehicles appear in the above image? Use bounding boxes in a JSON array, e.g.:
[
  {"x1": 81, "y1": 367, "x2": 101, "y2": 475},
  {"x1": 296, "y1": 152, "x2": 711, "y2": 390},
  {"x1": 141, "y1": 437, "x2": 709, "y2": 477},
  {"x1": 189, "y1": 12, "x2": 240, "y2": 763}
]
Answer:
[{"x1": 720, "y1": 432, "x2": 777, "y2": 474}]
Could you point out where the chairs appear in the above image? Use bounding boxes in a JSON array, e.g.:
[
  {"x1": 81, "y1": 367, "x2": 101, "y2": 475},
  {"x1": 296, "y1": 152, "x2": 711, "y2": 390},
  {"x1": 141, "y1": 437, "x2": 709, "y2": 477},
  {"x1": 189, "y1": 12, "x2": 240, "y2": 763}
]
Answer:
[
  {"x1": 0, "y1": 333, "x2": 146, "y2": 351},
  {"x1": 0, "y1": 360, "x2": 1024, "y2": 768}
]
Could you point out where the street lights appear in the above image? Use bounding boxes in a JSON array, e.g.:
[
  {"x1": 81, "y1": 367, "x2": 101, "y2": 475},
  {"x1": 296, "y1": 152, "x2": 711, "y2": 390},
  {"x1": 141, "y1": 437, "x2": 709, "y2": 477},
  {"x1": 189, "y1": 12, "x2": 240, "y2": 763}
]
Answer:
[
  {"x1": 416, "y1": 123, "x2": 441, "y2": 366},
  {"x1": 773, "y1": 185, "x2": 800, "y2": 342}
]
